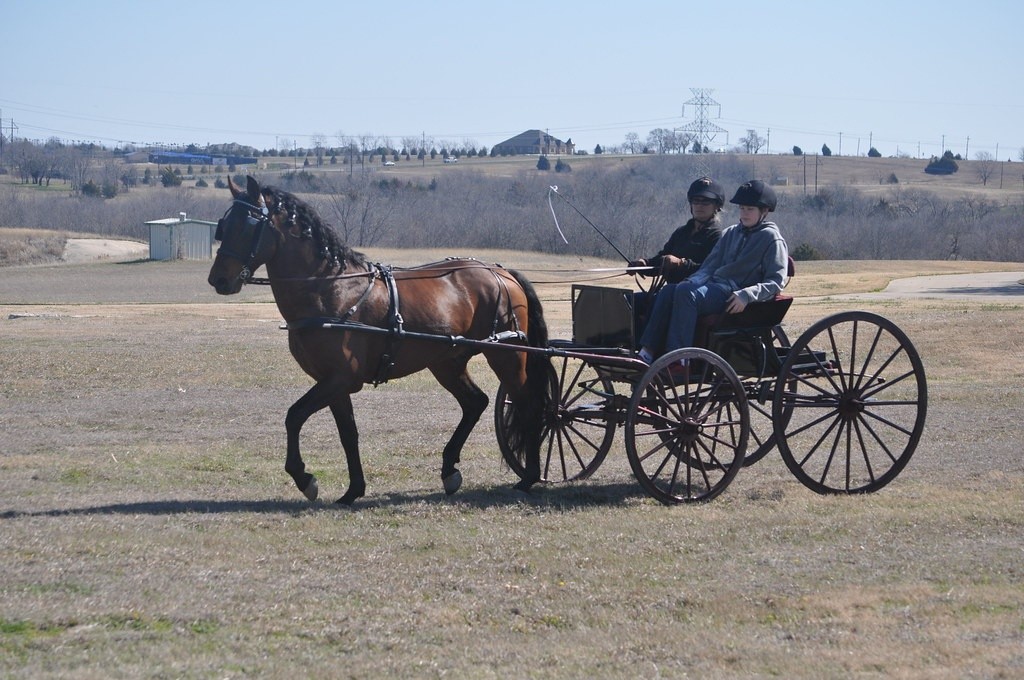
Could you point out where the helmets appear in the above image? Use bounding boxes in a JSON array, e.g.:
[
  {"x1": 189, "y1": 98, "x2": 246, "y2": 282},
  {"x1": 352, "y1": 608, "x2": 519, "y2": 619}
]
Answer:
[
  {"x1": 730, "y1": 179, "x2": 777, "y2": 212},
  {"x1": 687, "y1": 176, "x2": 725, "y2": 206}
]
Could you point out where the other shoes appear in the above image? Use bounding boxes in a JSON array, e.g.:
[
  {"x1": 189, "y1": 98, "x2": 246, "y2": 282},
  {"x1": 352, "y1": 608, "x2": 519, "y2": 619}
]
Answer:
[
  {"x1": 633, "y1": 355, "x2": 645, "y2": 361},
  {"x1": 666, "y1": 362, "x2": 692, "y2": 375}
]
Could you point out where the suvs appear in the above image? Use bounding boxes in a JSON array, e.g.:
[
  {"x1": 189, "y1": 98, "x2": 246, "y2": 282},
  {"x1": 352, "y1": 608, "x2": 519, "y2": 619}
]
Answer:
[{"x1": 444, "y1": 157, "x2": 458, "y2": 162}]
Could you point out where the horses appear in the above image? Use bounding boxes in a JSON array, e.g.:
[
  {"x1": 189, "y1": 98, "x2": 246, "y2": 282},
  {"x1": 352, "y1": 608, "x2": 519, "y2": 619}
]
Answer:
[{"x1": 207, "y1": 174, "x2": 551, "y2": 506}]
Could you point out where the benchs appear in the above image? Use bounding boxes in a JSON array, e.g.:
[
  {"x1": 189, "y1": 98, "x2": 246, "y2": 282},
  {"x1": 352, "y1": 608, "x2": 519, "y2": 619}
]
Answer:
[{"x1": 693, "y1": 255, "x2": 795, "y2": 348}]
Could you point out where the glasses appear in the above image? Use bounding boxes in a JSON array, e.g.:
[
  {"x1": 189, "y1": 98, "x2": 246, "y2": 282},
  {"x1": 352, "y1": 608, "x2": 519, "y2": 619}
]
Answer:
[{"x1": 691, "y1": 199, "x2": 712, "y2": 205}]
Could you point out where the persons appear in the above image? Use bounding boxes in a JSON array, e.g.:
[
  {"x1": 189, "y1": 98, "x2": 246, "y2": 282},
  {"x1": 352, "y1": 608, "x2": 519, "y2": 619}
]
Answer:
[
  {"x1": 633, "y1": 180, "x2": 789, "y2": 376},
  {"x1": 627, "y1": 176, "x2": 726, "y2": 336}
]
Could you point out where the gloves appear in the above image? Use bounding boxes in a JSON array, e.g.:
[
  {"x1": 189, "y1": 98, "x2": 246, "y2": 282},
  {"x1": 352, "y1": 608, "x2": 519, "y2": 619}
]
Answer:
[
  {"x1": 659, "y1": 255, "x2": 681, "y2": 271},
  {"x1": 627, "y1": 258, "x2": 646, "y2": 275}
]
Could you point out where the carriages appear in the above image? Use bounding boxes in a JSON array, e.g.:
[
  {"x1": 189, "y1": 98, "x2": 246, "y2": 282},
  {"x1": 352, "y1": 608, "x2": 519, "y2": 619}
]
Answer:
[{"x1": 208, "y1": 175, "x2": 928, "y2": 507}]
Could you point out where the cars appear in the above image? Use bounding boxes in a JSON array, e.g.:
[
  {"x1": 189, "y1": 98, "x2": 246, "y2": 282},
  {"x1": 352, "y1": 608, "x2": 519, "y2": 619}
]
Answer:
[{"x1": 383, "y1": 161, "x2": 395, "y2": 166}]
